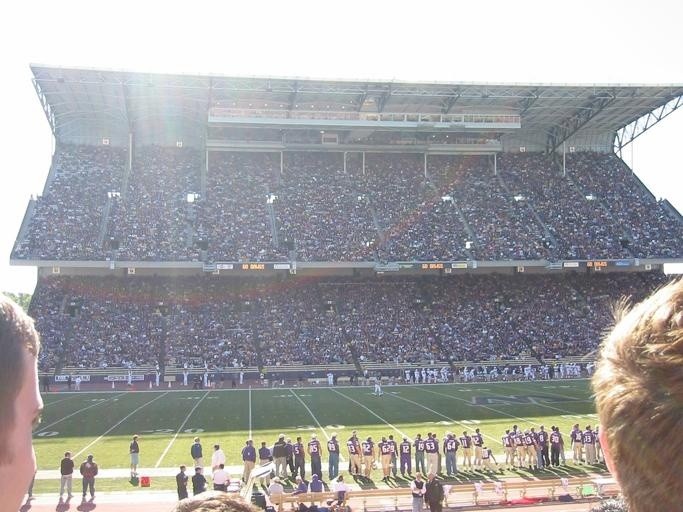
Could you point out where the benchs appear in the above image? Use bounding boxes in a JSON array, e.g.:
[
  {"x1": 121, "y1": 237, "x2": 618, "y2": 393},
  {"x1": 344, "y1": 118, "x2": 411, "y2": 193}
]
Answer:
[{"x1": 305, "y1": 374, "x2": 392, "y2": 386}]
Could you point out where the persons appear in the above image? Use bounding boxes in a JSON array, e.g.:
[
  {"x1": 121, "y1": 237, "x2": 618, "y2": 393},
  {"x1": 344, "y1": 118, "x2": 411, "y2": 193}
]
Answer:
[
  {"x1": 80, "y1": 455, "x2": 98, "y2": 498},
  {"x1": 59, "y1": 451, "x2": 75, "y2": 498},
  {"x1": 10, "y1": 132, "x2": 683, "y2": 394},
  {"x1": 129, "y1": 434, "x2": 140, "y2": 477},
  {"x1": 0, "y1": 294, "x2": 42, "y2": 512},
  {"x1": 28, "y1": 467, "x2": 38, "y2": 502},
  {"x1": 589, "y1": 273, "x2": 682, "y2": 512},
  {"x1": 171, "y1": 424, "x2": 606, "y2": 512}
]
